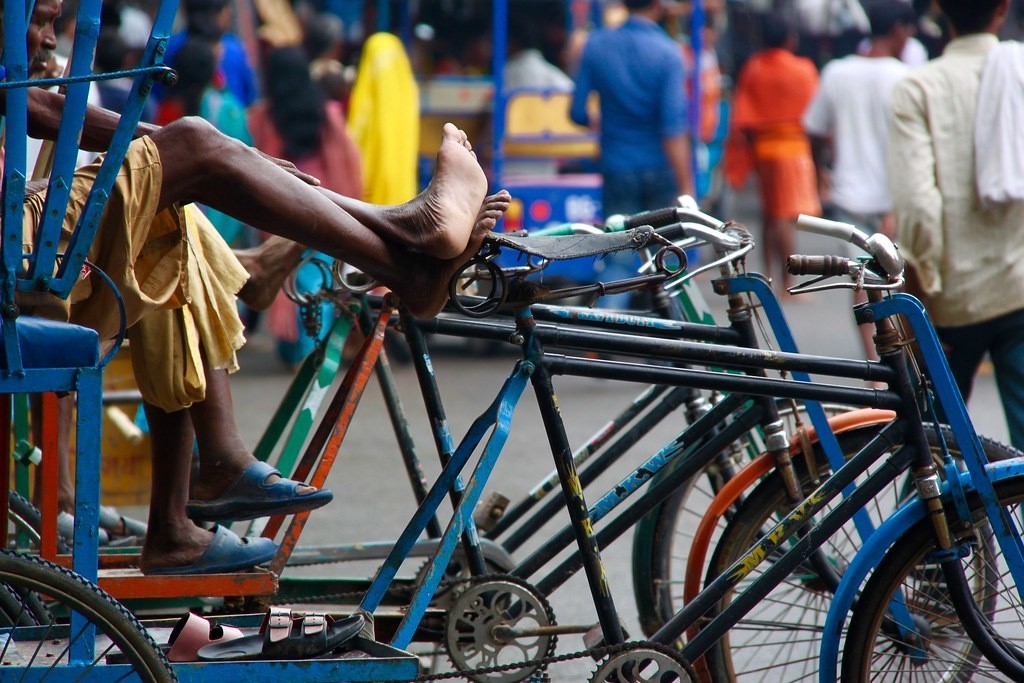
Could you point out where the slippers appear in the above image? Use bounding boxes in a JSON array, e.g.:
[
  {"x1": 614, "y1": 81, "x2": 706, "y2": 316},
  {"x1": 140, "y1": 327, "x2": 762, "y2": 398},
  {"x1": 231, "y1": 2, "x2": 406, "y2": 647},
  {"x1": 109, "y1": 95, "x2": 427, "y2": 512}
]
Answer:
[
  {"x1": 105, "y1": 608, "x2": 243, "y2": 663},
  {"x1": 145, "y1": 522, "x2": 279, "y2": 576},
  {"x1": 92, "y1": 505, "x2": 150, "y2": 548},
  {"x1": 196, "y1": 603, "x2": 364, "y2": 663},
  {"x1": 55, "y1": 512, "x2": 138, "y2": 553},
  {"x1": 186, "y1": 461, "x2": 334, "y2": 523}
]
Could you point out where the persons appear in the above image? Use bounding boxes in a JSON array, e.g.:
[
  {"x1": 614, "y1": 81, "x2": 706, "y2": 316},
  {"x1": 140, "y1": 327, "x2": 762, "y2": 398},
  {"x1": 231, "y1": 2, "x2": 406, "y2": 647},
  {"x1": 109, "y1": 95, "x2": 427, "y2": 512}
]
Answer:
[
  {"x1": 882, "y1": 0, "x2": 1024, "y2": 661},
  {"x1": 0, "y1": 0, "x2": 513, "y2": 578},
  {"x1": 547, "y1": 0, "x2": 954, "y2": 391}
]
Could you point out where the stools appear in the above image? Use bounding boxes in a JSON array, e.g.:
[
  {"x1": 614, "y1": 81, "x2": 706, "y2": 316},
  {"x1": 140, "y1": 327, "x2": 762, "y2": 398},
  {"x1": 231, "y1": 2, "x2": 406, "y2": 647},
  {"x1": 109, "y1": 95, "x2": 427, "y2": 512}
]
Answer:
[{"x1": 0, "y1": 307, "x2": 105, "y2": 396}]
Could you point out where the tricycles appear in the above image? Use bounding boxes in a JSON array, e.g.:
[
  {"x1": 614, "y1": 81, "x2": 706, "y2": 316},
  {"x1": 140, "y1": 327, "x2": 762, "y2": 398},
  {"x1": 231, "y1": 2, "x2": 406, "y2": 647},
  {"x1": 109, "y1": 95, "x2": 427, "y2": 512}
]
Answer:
[{"x1": 0, "y1": 1, "x2": 1024, "y2": 683}]
